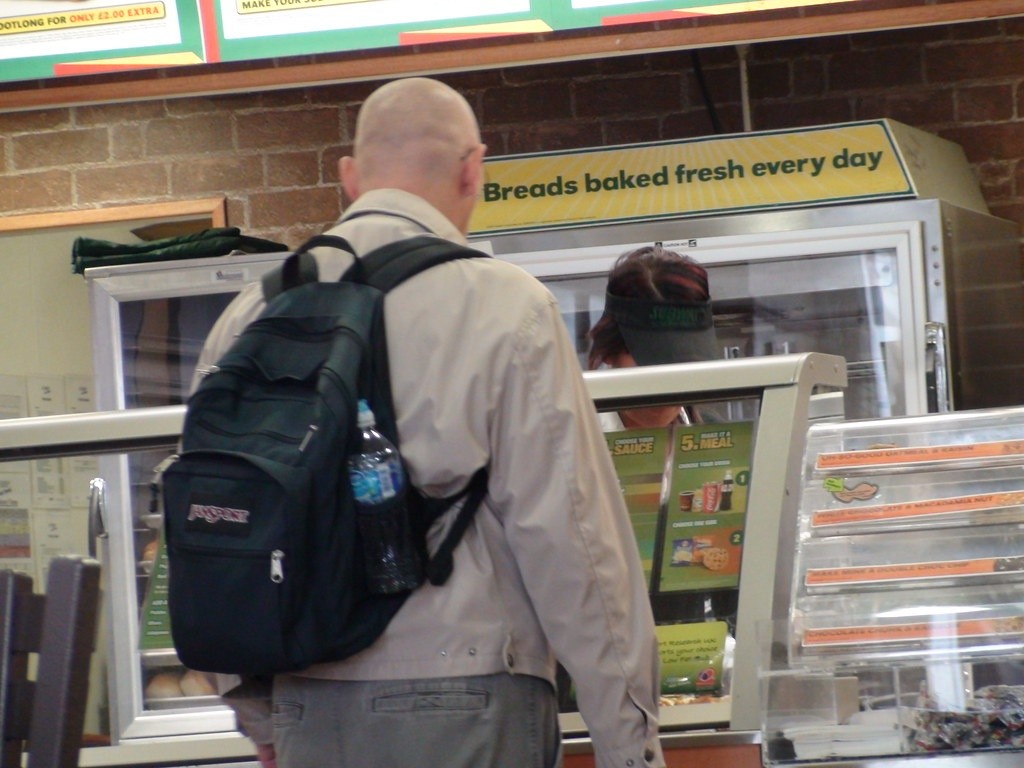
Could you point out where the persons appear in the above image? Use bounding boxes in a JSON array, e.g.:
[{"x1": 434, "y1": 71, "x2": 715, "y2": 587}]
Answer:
[
  {"x1": 556, "y1": 246, "x2": 738, "y2": 717},
  {"x1": 190, "y1": 77, "x2": 667, "y2": 768}
]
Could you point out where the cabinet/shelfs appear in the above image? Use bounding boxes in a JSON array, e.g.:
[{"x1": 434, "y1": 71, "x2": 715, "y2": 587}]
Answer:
[{"x1": 81, "y1": 252, "x2": 296, "y2": 744}]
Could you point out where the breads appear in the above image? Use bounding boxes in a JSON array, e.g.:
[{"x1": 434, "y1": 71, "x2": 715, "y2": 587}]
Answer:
[
  {"x1": 145, "y1": 670, "x2": 217, "y2": 699},
  {"x1": 702, "y1": 547, "x2": 728, "y2": 571},
  {"x1": 143, "y1": 540, "x2": 158, "y2": 572}
]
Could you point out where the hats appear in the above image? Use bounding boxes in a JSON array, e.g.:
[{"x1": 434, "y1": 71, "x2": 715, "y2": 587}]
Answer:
[{"x1": 603, "y1": 292, "x2": 720, "y2": 367}]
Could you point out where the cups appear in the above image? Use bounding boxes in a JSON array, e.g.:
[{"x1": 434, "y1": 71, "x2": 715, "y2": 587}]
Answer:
[
  {"x1": 679, "y1": 490, "x2": 694, "y2": 511},
  {"x1": 701, "y1": 483, "x2": 721, "y2": 513}
]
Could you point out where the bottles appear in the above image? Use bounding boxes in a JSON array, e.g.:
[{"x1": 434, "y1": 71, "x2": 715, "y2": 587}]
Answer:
[
  {"x1": 348, "y1": 397, "x2": 421, "y2": 597},
  {"x1": 719, "y1": 469, "x2": 734, "y2": 510}
]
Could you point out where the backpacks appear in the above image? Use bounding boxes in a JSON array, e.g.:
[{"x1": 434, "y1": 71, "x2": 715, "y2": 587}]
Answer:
[{"x1": 160, "y1": 236, "x2": 492, "y2": 676}]
